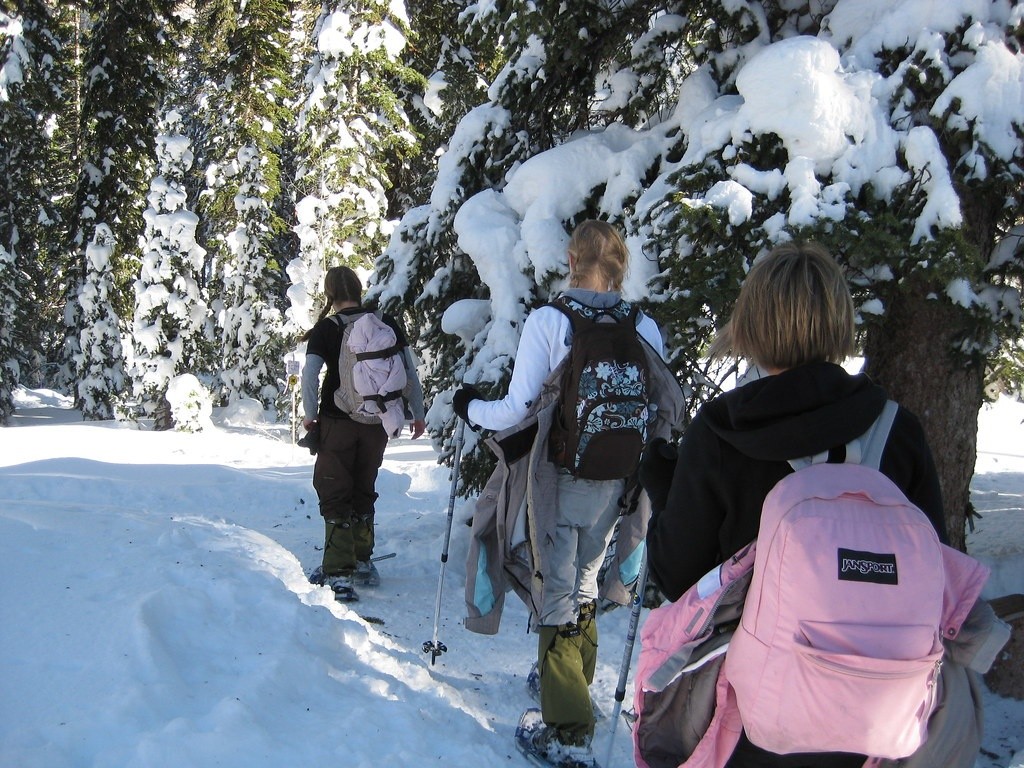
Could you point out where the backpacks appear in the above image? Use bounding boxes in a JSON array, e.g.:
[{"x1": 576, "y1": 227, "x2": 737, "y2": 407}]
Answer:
[
  {"x1": 725, "y1": 401, "x2": 943, "y2": 758},
  {"x1": 334, "y1": 309, "x2": 415, "y2": 424},
  {"x1": 543, "y1": 295, "x2": 661, "y2": 481}
]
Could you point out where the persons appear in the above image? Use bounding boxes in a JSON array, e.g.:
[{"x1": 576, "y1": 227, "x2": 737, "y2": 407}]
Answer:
[
  {"x1": 633, "y1": 241, "x2": 1012, "y2": 768},
  {"x1": 297, "y1": 265, "x2": 426, "y2": 602},
  {"x1": 453, "y1": 220, "x2": 687, "y2": 768}
]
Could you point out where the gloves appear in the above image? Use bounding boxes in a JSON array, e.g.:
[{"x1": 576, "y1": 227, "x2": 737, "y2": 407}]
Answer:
[{"x1": 453, "y1": 383, "x2": 482, "y2": 432}]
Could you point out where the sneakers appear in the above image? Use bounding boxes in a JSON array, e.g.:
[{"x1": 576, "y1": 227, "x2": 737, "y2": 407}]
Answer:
[
  {"x1": 515, "y1": 708, "x2": 600, "y2": 768},
  {"x1": 526, "y1": 661, "x2": 541, "y2": 705},
  {"x1": 352, "y1": 553, "x2": 396, "y2": 586},
  {"x1": 309, "y1": 565, "x2": 359, "y2": 601}
]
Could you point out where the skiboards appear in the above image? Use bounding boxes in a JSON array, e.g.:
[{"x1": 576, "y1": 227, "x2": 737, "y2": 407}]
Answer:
[
  {"x1": 307, "y1": 558, "x2": 381, "y2": 603},
  {"x1": 516, "y1": 658, "x2": 608, "y2": 768}
]
